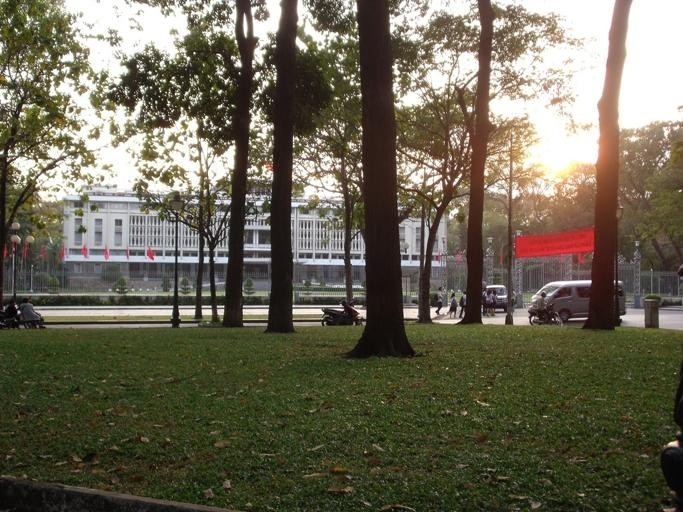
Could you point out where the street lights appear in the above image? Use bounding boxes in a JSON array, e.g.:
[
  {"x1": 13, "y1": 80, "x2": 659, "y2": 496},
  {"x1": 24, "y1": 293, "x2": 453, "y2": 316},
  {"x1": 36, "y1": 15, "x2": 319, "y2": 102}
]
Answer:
[
  {"x1": 614, "y1": 195, "x2": 625, "y2": 328},
  {"x1": 5, "y1": 221, "x2": 35, "y2": 303},
  {"x1": 165, "y1": 191, "x2": 186, "y2": 328}
]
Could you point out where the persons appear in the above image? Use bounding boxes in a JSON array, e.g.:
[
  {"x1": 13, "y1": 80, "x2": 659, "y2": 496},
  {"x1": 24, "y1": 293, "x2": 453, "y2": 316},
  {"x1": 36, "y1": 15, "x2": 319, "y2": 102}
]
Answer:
[
  {"x1": 534, "y1": 291, "x2": 552, "y2": 326},
  {"x1": 434, "y1": 286, "x2": 497, "y2": 318}
]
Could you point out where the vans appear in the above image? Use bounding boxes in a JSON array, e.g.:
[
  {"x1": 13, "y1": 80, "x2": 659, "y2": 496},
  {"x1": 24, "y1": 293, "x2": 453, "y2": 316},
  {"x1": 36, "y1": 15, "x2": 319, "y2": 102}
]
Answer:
[{"x1": 530, "y1": 279, "x2": 629, "y2": 318}]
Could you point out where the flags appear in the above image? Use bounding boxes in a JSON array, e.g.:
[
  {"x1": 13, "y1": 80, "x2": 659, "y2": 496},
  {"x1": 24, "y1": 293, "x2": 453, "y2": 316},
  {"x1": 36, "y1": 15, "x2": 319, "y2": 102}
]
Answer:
[
  {"x1": 3, "y1": 241, "x2": 7, "y2": 258},
  {"x1": 126, "y1": 246, "x2": 129, "y2": 261},
  {"x1": 81, "y1": 242, "x2": 87, "y2": 258},
  {"x1": 11, "y1": 240, "x2": 16, "y2": 255},
  {"x1": 23, "y1": 240, "x2": 27, "y2": 257},
  {"x1": 104, "y1": 244, "x2": 109, "y2": 259},
  {"x1": 40, "y1": 241, "x2": 46, "y2": 257},
  {"x1": 148, "y1": 244, "x2": 154, "y2": 260},
  {"x1": 58, "y1": 245, "x2": 63, "y2": 261}
]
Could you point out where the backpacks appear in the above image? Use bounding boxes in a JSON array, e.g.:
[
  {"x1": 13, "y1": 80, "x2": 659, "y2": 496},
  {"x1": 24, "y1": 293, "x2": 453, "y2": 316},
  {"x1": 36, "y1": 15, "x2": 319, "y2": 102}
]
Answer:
[
  {"x1": 481, "y1": 296, "x2": 497, "y2": 306},
  {"x1": 459, "y1": 296, "x2": 463, "y2": 306},
  {"x1": 434, "y1": 294, "x2": 438, "y2": 300}
]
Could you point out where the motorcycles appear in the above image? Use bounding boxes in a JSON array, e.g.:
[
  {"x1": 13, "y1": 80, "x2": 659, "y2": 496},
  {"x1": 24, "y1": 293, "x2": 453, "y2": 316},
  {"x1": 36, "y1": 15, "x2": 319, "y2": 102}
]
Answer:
[
  {"x1": 525, "y1": 304, "x2": 561, "y2": 327},
  {"x1": 319, "y1": 296, "x2": 367, "y2": 326}
]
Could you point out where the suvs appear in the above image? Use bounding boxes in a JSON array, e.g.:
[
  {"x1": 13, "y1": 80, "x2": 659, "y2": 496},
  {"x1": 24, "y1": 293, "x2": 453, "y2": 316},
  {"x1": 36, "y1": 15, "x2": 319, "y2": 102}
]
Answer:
[{"x1": 481, "y1": 283, "x2": 512, "y2": 314}]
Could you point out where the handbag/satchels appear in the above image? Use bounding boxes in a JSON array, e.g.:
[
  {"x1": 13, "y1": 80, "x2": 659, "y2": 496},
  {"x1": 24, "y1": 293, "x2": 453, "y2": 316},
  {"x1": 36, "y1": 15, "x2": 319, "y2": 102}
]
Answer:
[{"x1": 452, "y1": 303, "x2": 457, "y2": 307}]
